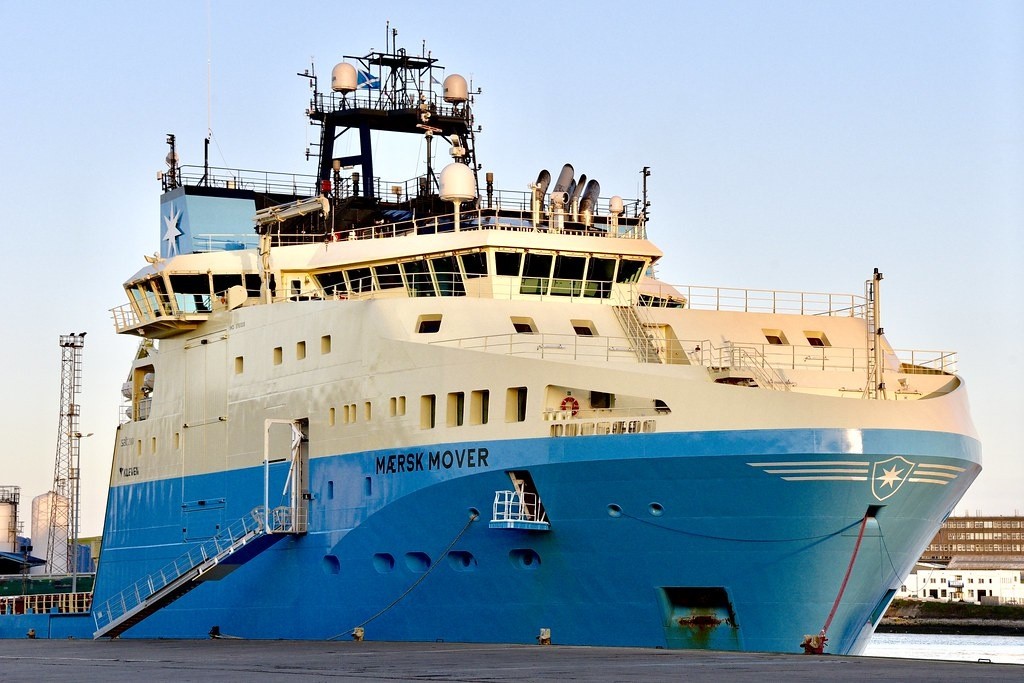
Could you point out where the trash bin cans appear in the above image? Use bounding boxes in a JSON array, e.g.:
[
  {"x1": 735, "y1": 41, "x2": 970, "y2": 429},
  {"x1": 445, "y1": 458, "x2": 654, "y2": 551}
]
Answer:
[{"x1": 1019, "y1": 598, "x2": 1024, "y2": 604}]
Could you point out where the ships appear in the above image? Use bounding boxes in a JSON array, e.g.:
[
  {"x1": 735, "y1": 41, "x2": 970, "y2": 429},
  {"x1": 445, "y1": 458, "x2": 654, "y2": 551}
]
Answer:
[{"x1": 0, "y1": 16, "x2": 986, "y2": 660}]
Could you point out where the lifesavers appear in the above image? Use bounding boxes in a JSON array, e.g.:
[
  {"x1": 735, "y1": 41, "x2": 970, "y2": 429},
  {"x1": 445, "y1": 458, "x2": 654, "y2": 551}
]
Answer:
[{"x1": 561, "y1": 396, "x2": 579, "y2": 416}]
